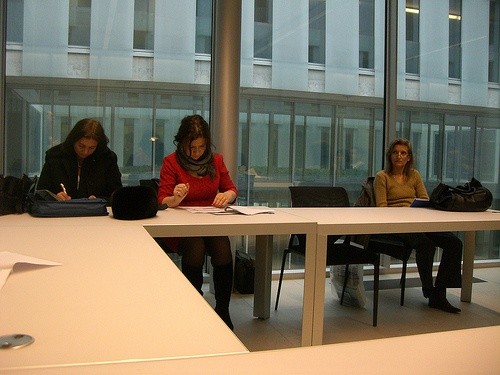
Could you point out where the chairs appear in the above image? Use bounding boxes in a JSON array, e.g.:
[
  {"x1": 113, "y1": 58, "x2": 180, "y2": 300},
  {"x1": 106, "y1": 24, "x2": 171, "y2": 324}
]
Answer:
[{"x1": 275, "y1": 176, "x2": 412, "y2": 327}]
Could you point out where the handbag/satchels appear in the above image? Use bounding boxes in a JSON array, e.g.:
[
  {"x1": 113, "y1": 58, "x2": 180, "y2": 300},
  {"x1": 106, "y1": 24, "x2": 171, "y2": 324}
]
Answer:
[
  {"x1": 430, "y1": 177, "x2": 493, "y2": 212},
  {"x1": 333, "y1": 265, "x2": 366, "y2": 306},
  {"x1": 0, "y1": 173, "x2": 110, "y2": 217},
  {"x1": 234, "y1": 249, "x2": 255, "y2": 294},
  {"x1": 111, "y1": 186, "x2": 168, "y2": 220}
]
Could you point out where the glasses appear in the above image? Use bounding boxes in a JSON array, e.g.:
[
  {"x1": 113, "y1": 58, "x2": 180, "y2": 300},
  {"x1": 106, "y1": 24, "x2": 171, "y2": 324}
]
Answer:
[{"x1": 392, "y1": 150, "x2": 409, "y2": 157}]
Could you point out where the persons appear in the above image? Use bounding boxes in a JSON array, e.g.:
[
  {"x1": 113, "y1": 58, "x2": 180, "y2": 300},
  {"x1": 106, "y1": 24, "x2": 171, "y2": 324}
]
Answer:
[
  {"x1": 37, "y1": 119, "x2": 123, "y2": 201},
  {"x1": 373, "y1": 138, "x2": 462, "y2": 313},
  {"x1": 158, "y1": 114, "x2": 238, "y2": 331}
]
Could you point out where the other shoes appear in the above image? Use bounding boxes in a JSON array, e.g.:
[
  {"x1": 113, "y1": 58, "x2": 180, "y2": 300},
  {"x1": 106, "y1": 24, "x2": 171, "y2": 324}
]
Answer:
[
  {"x1": 215, "y1": 304, "x2": 233, "y2": 331},
  {"x1": 422, "y1": 277, "x2": 433, "y2": 299},
  {"x1": 428, "y1": 295, "x2": 461, "y2": 312}
]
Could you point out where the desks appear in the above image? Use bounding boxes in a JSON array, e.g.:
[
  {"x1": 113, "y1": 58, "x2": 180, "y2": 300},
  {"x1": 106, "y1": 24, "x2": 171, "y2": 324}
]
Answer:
[{"x1": 0, "y1": 207, "x2": 500, "y2": 375}]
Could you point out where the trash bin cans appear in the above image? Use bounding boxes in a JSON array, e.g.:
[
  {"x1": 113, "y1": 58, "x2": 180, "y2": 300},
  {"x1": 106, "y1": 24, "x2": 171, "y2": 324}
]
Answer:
[{"x1": 235, "y1": 250, "x2": 254, "y2": 294}]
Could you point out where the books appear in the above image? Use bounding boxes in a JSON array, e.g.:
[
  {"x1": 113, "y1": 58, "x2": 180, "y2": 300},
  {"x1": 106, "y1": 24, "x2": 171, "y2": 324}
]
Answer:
[{"x1": 410, "y1": 198, "x2": 429, "y2": 207}]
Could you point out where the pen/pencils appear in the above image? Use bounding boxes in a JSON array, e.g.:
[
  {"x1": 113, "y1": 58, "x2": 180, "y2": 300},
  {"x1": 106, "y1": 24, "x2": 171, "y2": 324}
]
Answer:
[{"x1": 60, "y1": 183, "x2": 67, "y2": 194}]
